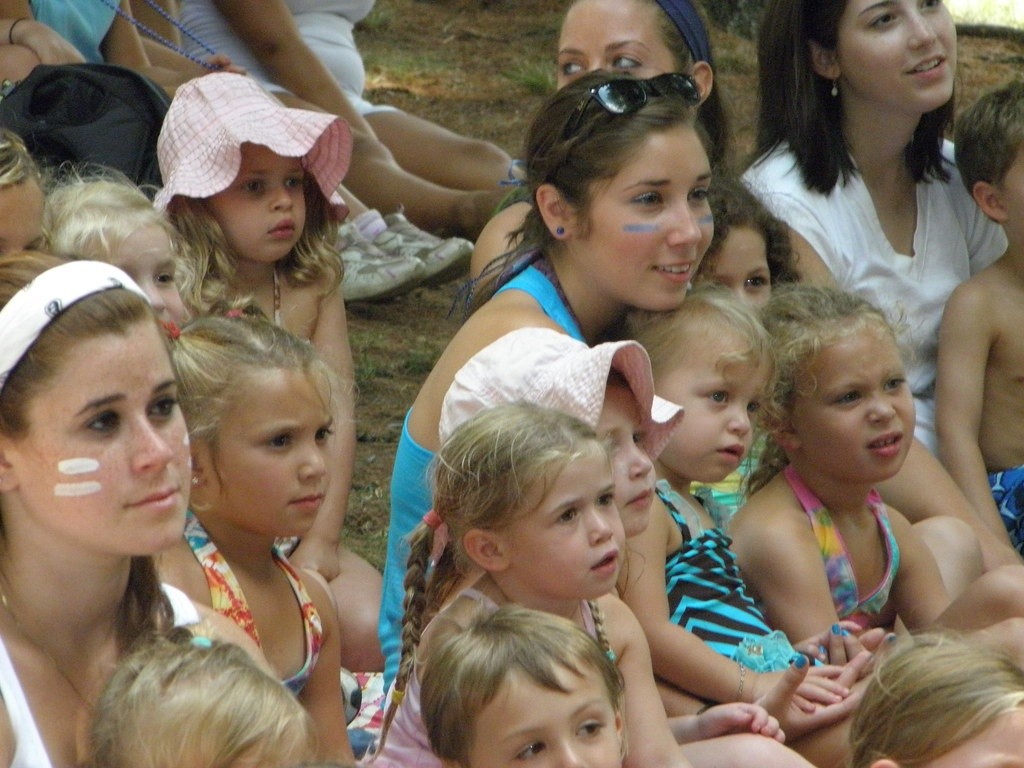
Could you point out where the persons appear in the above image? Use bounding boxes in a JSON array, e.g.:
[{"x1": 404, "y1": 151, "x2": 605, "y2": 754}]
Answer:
[
  {"x1": 0, "y1": 250, "x2": 885, "y2": 768},
  {"x1": 473, "y1": 0, "x2": 1024, "y2": 767},
  {"x1": 0, "y1": 0, "x2": 528, "y2": 669}
]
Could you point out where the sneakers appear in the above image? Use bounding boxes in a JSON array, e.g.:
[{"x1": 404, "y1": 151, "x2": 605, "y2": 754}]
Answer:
[
  {"x1": 329, "y1": 222, "x2": 429, "y2": 305},
  {"x1": 368, "y1": 202, "x2": 475, "y2": 290}
]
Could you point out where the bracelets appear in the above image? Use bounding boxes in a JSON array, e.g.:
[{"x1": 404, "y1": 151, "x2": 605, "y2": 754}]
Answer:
[
  {"x1": 9, "y1": 17, "x2": 26, "y2": 44},
  {"x1": 735, "y1": 662, "x2": 746, "y2": 700}
]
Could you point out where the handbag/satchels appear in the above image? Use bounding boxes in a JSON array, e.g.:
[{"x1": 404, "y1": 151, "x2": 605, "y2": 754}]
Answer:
[{"x1": 0, "y1": 62, "x2": 171, "y2": 205}]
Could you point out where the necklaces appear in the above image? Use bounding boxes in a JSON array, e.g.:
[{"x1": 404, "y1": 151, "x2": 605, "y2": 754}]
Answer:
[{"x1": 96, "y1": 0, "x2": 221, "y2": 69}]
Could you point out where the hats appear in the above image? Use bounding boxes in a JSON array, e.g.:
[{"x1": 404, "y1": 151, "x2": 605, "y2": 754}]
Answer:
[
  {"x1": 150, "y1": 71, "x2": 353, "y2": 222},
  {"x1": 438, "y1": 326, "x2": 686, "y2": 464}
]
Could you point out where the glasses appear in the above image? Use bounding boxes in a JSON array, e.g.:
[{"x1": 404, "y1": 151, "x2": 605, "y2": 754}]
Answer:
[{"x1": 547, "y1": 72, "x2": 701, "y2": 182}]
